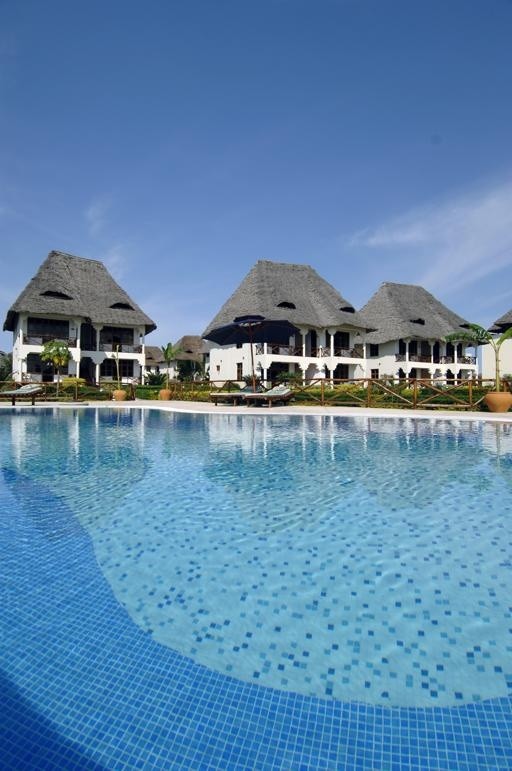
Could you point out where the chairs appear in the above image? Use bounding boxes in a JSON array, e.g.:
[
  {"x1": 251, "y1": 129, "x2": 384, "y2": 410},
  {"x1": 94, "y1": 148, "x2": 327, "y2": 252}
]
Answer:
[
  {"x1": 210, "y1": 384, "x2": 293, "y2": 406},
  {"x1": 0, "y1": 382, "x2": 45, "y2": 405}
]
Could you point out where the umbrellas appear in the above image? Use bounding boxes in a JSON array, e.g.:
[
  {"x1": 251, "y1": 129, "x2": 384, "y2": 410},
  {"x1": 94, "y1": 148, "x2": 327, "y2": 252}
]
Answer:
[{"x1": 200, "y1": 313, "x2": 301, "y2": 404}]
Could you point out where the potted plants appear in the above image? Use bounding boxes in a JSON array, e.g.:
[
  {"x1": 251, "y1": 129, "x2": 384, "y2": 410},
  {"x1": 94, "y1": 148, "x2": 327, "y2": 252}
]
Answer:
[
  {"x1": 159, "y1": 343, "x2": 184, "y2": 400},
  {"x1": 112, "y1": 345, "x2": 128, "y2": 401},
  {"x1": 442, "y1": 323, "x2": 512, "y2": 412}
]
